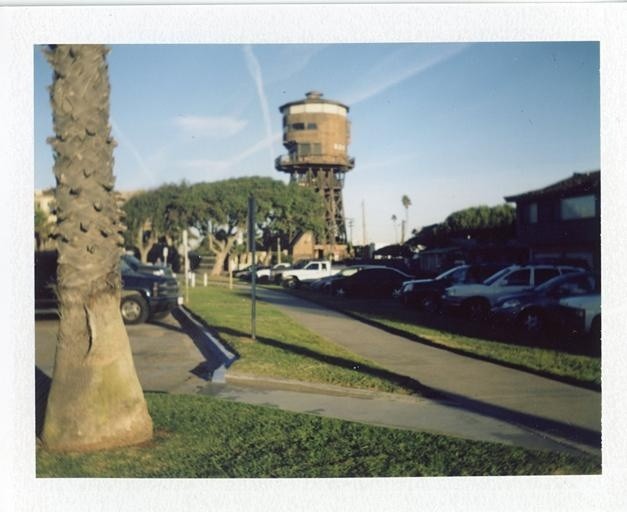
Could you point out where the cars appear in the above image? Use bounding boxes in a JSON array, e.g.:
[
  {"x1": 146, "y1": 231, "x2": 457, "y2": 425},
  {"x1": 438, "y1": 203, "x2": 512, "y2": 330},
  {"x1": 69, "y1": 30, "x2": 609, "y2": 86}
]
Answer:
[
  {"x1": 34, "y1": 247, "x2": 181, "y2": 325},
  {"x1": 312, "y1": 262, "x2": 603, "y2": 343},
  {"x1": 231, "y1": 263, "x2": 292, "y2": 284}
]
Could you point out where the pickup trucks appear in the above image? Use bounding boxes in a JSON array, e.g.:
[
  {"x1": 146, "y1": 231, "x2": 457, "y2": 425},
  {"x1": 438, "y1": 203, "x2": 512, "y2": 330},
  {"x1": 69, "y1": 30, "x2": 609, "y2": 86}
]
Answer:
[{"x1": 275, "y1": 261, "x2": 361, "y2": 290}]
